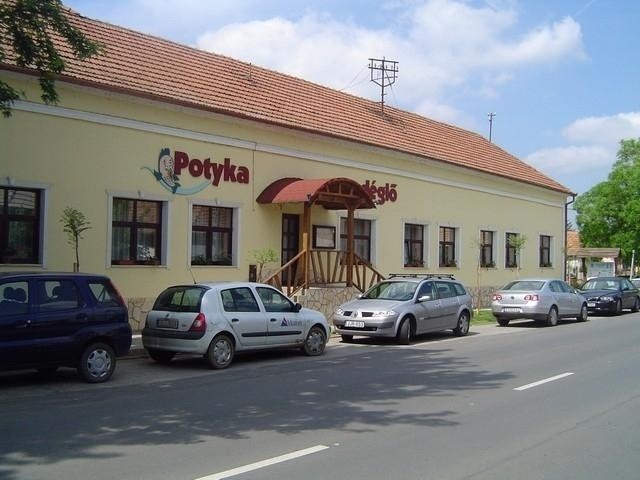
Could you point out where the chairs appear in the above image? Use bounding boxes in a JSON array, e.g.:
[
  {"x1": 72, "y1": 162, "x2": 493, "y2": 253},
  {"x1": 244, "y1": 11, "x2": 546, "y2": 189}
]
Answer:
[
  {"x1": 0, "y1": 286, "x2": 28, "y2": 315},
  {"x1": 47, "y1": 286, "x2": 66, "y2": 304}
]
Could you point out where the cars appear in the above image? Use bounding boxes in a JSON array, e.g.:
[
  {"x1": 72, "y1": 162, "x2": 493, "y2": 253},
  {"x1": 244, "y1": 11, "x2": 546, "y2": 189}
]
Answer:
[
  {"x1": 142, "y1": 281, "x2": 331, "y2": 369},
  {"x1": 0, "y1": 272, "x2": 132, "y2": 383},
  {"x1": 333, "y1": 274, "x2": 472, "y2": 344},
  {"x1": 491, "y1": 277, "x2": 640, "y2": 326}
]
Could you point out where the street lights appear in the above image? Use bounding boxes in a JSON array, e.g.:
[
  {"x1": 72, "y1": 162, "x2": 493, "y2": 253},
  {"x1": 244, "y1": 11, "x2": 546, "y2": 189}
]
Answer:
[{"x1": 488, "y1": 112, "x2": 496, "y2": 142}]
